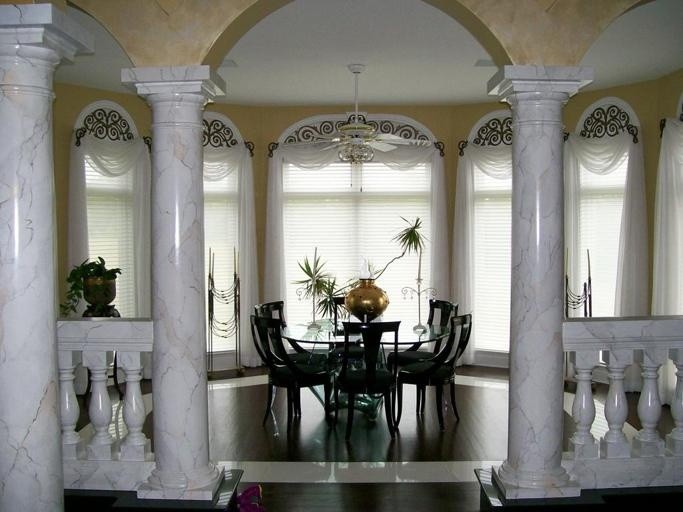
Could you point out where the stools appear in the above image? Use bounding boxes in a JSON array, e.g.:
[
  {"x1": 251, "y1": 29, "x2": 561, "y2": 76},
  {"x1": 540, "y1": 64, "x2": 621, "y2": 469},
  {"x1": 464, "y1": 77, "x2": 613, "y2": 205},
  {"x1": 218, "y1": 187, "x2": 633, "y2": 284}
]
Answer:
[{"x1": 82, "y1": 305, "x2": 124, "y2": 407}]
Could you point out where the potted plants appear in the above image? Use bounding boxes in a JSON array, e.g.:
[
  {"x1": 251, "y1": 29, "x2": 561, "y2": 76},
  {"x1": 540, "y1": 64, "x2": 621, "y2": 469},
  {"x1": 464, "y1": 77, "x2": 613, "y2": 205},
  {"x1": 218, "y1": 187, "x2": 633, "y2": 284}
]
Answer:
[{"x1": 59, "y1": 256, "x2": 122, "y2": 317}]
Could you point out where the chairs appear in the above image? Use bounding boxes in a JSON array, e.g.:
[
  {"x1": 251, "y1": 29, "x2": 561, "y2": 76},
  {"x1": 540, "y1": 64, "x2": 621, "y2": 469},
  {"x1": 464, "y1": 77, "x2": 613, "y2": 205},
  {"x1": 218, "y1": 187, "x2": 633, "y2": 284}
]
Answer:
[
  {"x1": 255, "y1": 301, "x2": 325, "y2": 417},
  {"x1": 395, "y1": 314, "x2": 472, "y2": 429},
  {"x1": 334, "y1": 320, "x2": 401, "y2": 439},
  {"x1": 332, "y1": 297, "x2": 345, "y2": 317},
  {"x1": 250, "y1": 314, "x2": 334, "y2": 441},
  {"x1": 387, "y1": 299, "x2": 460, "y2": 416}
]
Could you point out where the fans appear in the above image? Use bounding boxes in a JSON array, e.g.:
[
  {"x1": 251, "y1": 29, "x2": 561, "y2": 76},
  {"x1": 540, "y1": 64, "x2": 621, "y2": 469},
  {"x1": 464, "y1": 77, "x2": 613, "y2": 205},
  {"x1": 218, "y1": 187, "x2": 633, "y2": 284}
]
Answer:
[{"x1": 281, "y1": 64, "x2": 431, "y2": 170}]
